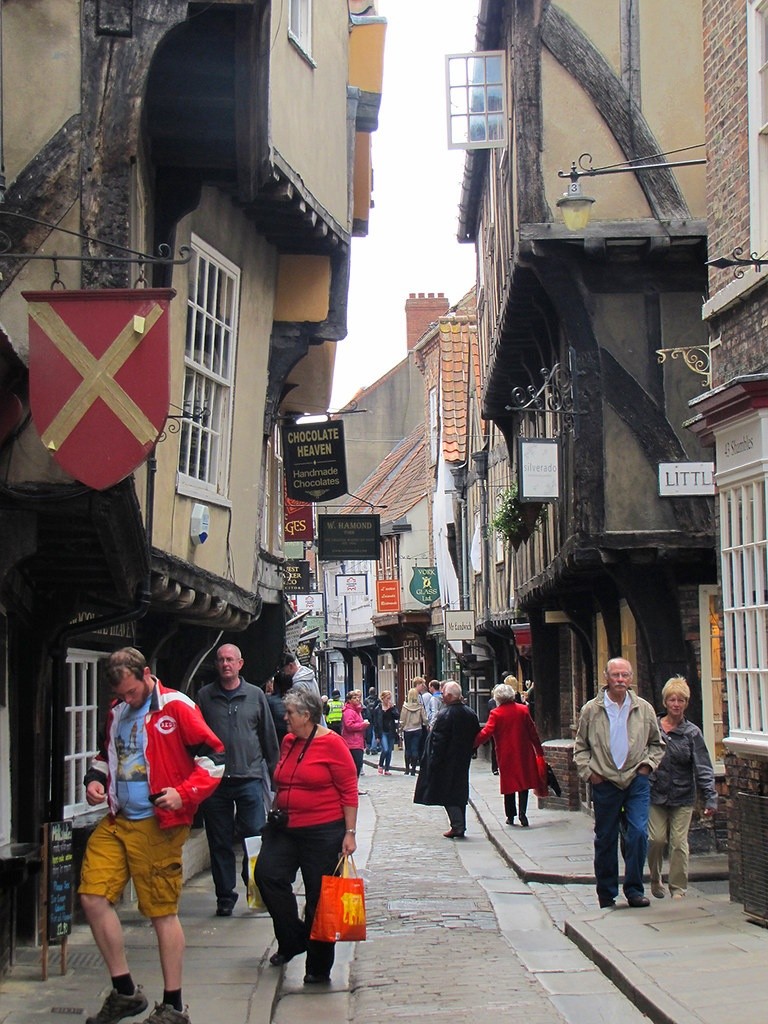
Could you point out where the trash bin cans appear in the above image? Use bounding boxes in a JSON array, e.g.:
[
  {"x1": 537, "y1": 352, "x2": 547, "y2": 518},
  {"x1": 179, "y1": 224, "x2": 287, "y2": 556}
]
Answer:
[{"x1": 739, "y1": 790, "x2": 768, "y2": 917}]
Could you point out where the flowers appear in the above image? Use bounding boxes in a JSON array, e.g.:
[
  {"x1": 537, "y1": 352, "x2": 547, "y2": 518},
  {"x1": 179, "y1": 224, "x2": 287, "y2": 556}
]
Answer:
[{"x1": 481, "y1": 476, "x2": 547, "y2": 550}]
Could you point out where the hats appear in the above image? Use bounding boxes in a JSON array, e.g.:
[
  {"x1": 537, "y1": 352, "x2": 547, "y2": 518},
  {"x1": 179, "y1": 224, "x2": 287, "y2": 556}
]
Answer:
[{"x1": 282, "y1": 653, "x2": 296, "y2": 666}]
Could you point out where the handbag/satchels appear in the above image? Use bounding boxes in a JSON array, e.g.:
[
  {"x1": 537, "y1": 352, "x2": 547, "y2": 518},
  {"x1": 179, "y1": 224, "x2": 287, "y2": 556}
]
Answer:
[
  {"x1": 245, "y1": 836, "x2": 268, "y2": 913},
  {"x1": 310, "y1": 851, "x2": 367, "y2": 942},
  {"x1": 533, "y1": 755, "x2": 561, "y2": 797}
]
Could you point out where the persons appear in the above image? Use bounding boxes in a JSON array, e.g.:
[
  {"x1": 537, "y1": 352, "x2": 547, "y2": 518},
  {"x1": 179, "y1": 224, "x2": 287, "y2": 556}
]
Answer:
[
  {"x1": 473, "y1": 684, "x2": 545, "y2": 826},
  {"x1": 264, "y1": 652, "x2": 328, "y2": 746},
  {"x1": 253, "y1": 686, "x2": 358, "y2": 984},
  {"x1": 322, "y1": 677, "x2": 447, "y2": 795},
  {"x1": 373, "y1": 690, "x2": 400, "y2": 776},
  {"x1": 76, "y1": 647, "x2": 226, "y2": 1024},
  {"x1": 413, "y1": 681, "x2": 480, "y2": 837},
  {"x1": 196, "y1": 642, "x2": 280, "y2": 917},
  {"x1": 487, "y1": 675, "x2": 534, "y2": 775},
  {"x1": 573, "y1": 658, "x2": 666, "y2": 909},
  {"x1": 647, "y1": 673, "x2": 719, "y2": 898}
]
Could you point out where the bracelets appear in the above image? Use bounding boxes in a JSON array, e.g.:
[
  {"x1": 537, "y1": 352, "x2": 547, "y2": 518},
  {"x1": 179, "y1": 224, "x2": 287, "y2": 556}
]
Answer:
[{"x1": 345, "y1": 829, "x2": 356, "y2": 833}]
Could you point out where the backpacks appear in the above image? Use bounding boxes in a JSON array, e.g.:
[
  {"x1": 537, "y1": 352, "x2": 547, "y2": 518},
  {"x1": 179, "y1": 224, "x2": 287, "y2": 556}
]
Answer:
[{"x1": 365, "y1": 698, "x2": 378, "y2": 723}]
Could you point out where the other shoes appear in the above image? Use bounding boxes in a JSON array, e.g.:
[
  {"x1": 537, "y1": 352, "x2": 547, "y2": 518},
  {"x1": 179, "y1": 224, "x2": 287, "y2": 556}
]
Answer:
[
  {"x1": 304, "y1": 974, "x2": 329, "y2": 983},
  {"x1": 216, "y1": 904, "x2": 232, "y2": 916},
  {"x1": 384, "y1": 772, "x2": 391, "y2": 775},
  {"x1": 671, "y1": 893, "x2": 682, "y2": 900},
  {"x1": 629, "y1": 897, "x2": 650, "y2": 907},
  {"x1": 270, "y1": 951, "x2": 294, "y2": 965},
  {"x1": 506, "y1": 818, "x2": 514, "y2": 825},
  {"x1": 378, "y1": 766, "x2": 383, "y2": 774},
  {"x1": 598, "y1": 896, "x2": 615, "y2": 908},
  {"x1": 443, "y1": 829, "x2": 464, "y2": 838},
  {"x1": 494, "y1": 771, "x2": 499, "y2": 775},
  {"x1": 651, "y1": 880, "x2": 666, "y2": 898},
  {"x1": 519, "y1": 814, "x2": 528, "y2": 826}
]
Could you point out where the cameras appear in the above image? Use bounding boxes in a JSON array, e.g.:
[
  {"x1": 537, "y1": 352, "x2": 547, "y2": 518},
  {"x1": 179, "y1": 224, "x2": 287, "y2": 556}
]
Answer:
[{"x1": 267, "y1": 809, "x2": 288, "y2": 828}]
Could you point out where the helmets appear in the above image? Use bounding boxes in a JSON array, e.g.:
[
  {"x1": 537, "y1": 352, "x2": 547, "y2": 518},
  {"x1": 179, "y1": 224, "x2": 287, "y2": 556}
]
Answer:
[{"x1": 331, "y1": 689, "x2": 340, "y2": 699}]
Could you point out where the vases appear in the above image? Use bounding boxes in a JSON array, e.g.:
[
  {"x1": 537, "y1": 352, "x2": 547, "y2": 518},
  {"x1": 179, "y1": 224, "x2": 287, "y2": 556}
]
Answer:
[
  {"x1": 509, "y1": 533, "x2": 522, "y2": 552},
  {"x1": 517, "y1": 526, "x2": 530, "y2": 545},
  {"x1": 510, "y1": 501, "x2": 543, "y2": 532}
]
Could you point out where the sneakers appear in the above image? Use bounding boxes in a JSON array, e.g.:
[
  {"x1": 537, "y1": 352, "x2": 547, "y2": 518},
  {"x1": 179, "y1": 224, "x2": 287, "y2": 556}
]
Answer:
[
  {"x1": 133, "y1": 1001, "x2": 191, "y2": 1024},
  {"x1": 86, "y1": 984, "x2": 148, "y2": 1024}
]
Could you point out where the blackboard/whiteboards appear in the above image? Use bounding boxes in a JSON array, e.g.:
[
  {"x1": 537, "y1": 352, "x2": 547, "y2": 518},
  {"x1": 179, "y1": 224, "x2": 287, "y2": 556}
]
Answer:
[{"x1": 46, "y1": 822, "x2": 73, "y2": 941}]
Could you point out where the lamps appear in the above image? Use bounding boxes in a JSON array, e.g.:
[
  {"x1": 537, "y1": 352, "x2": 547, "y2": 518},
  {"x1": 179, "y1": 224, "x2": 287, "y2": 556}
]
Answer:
[{"x1": 556, "y1": 143, "x2": 706, "y2": 230}]
